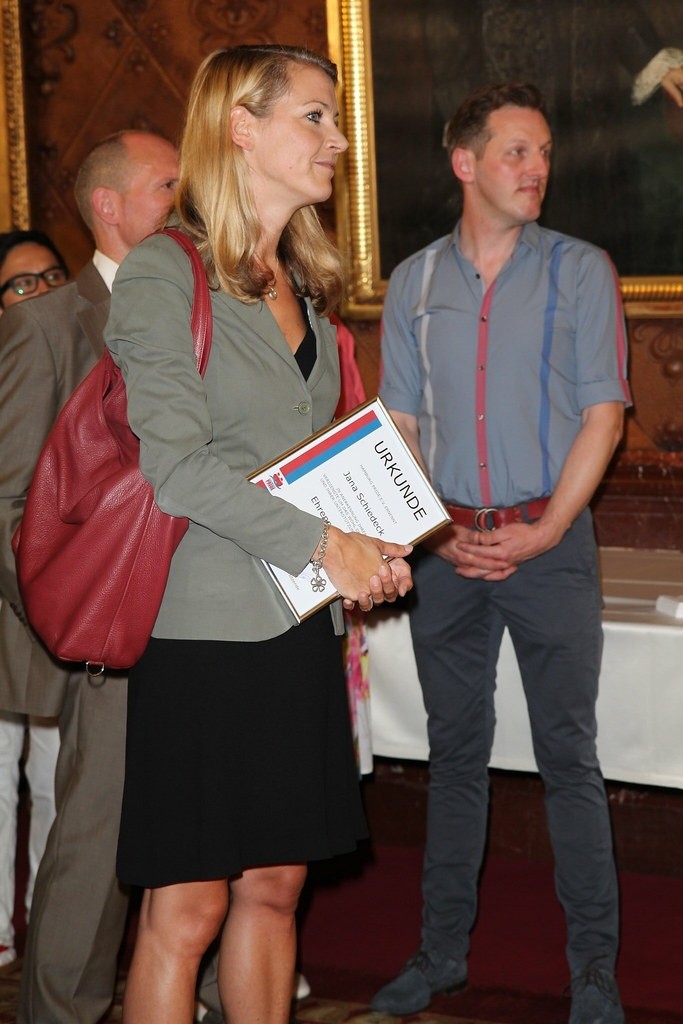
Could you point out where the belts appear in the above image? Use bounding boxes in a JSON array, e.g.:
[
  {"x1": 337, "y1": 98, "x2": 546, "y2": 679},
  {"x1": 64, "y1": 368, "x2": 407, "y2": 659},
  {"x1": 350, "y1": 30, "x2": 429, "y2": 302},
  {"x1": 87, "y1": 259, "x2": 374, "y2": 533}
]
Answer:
[{"x1": 446, "y1": 496, "x2": 553, "y2": 533}]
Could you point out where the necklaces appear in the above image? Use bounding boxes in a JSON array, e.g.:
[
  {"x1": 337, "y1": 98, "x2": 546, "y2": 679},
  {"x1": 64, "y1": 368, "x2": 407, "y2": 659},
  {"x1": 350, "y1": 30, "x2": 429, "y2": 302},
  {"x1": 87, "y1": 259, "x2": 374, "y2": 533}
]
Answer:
[{"x1": 265, "y1": 254, "x2": 279, "y2": 300}]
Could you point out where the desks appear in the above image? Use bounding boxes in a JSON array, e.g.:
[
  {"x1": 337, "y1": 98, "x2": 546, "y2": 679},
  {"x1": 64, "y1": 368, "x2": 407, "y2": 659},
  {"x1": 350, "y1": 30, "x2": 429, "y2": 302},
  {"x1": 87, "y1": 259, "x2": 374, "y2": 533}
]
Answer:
[{"x1": 357, "y1": 546, "x2": 681, "y2": 788}]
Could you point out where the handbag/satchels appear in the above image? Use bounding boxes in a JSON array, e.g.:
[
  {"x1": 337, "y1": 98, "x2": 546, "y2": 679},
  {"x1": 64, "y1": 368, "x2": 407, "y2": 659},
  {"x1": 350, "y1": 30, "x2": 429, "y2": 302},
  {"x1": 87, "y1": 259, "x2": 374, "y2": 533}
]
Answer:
[{"x1": 11, "y1": 229, "x2": 213, "y2": 676}]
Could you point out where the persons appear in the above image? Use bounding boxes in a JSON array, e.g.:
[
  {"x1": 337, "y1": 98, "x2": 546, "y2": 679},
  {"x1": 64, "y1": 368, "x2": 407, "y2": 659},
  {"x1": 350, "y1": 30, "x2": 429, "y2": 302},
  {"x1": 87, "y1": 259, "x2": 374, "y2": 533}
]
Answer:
[
  {"x1": 372, "y1": 83, "x2": 633, "y2": 1024},
  {"x1": 104, "y1": 44, "x2": 412, "y2": 1024},
  {"x1": 0, "y1": 126, "x2": 197, "y2": 1023},
  {"x1": 1, "y1": 230, "x2": 67, "y2": 971}
]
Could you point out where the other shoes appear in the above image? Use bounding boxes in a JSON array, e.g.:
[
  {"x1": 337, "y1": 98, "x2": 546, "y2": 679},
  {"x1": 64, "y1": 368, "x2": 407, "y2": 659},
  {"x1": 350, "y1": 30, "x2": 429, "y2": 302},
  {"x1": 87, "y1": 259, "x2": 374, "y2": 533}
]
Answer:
[
  {"x1": 291, "y1": 974, "x2": 310, "y2": 999},
  {"x1": 0, "y1": 945, "x2": 16, "y2": 966},
  {"x1": 193, "y1": 1000, "x2": 226, "y2": 1024}
]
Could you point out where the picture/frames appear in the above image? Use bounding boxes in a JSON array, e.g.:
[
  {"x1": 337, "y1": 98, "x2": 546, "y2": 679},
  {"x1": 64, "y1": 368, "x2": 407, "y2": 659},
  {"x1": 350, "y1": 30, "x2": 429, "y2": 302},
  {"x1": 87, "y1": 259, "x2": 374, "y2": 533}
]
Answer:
[{"x1": 3, "y1": 0, "x2": 683, "y2": 329}]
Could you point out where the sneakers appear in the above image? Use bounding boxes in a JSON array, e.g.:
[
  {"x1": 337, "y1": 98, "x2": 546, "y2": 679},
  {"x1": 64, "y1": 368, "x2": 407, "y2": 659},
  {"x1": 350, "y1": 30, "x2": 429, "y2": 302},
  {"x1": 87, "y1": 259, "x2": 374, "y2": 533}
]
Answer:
[
  {"x1": 569, "y1": 968, "x2": 624, "y2": 1024},
  {"x1": 373, "y1": 946, "x2": 468, "y2": 1014}
]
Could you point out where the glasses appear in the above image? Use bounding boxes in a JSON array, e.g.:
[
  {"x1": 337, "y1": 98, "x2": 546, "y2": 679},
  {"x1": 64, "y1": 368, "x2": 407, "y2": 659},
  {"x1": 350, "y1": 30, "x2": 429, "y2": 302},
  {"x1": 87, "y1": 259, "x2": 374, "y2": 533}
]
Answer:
[{"x1": 0, "y1": 265, "x2": 67, "y2": 303}]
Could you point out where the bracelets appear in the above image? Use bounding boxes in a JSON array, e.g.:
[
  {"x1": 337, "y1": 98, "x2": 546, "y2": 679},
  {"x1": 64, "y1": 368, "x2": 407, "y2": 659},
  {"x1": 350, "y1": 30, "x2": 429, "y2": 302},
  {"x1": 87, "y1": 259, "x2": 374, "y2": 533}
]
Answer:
[{"x1": 312, "y1": 521, "x2": 332, "y2": 591}]
